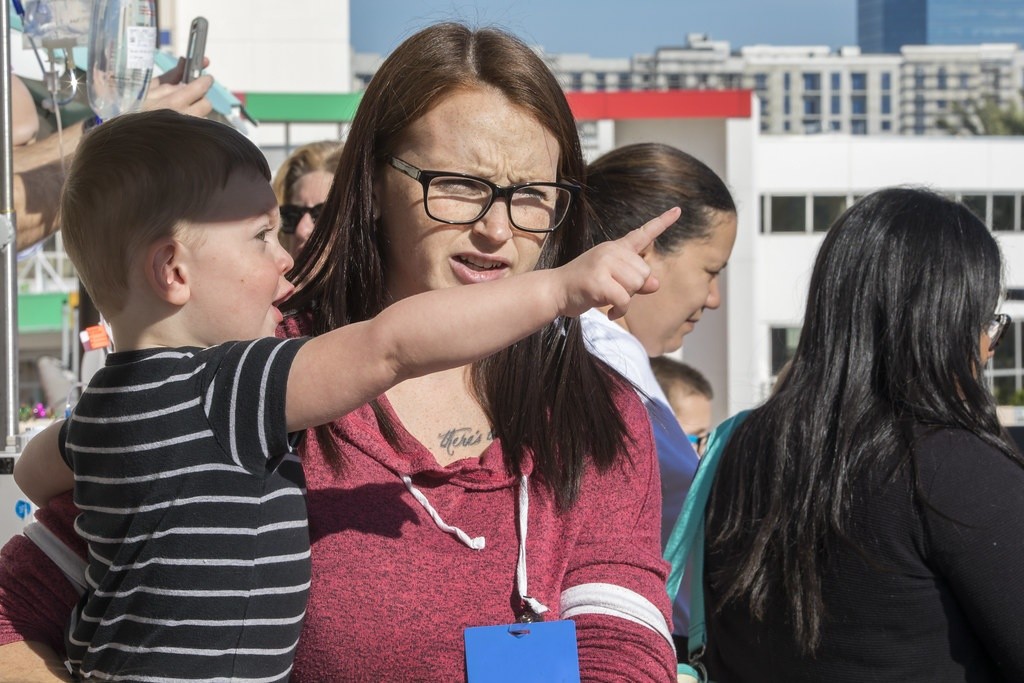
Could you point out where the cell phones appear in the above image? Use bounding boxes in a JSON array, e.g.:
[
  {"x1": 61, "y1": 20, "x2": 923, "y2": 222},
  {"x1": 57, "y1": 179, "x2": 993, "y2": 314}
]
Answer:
[{"x1": 181, "y1": 17, "x2": 209, "y2": 86}]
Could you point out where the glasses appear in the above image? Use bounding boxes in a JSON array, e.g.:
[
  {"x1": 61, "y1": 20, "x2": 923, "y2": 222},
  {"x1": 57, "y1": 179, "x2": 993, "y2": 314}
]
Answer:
[
  {"x1": 279, "y1": 204, "x2": 326, "y2": 235},
  {"x1": 688, "y1": 433, "x2": 709, "y2": 456},
  {"x1": 988, "y1": 313, "x2": 1011, "y2": 352},
  {"x1": 379, "y1": 153, "x2": 582, "y2": 233}
]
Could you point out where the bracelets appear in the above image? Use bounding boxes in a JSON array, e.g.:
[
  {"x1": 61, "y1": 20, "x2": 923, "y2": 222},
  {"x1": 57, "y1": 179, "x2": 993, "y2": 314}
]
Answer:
[{"x1": 81, "y1": 113, "x2": 103, "y2": 136}]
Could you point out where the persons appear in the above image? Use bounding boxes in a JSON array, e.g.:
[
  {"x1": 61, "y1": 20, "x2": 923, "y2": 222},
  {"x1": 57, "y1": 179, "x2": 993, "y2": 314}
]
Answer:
[
  {"x1": 8, "y1": 59, "x2": 346, "y2": 261},
  {"x1": 590, "y1": 142, "x2": 740, "y2": 634},
  {"x1": 13, "y1": 109, "x2": 681, "y2": 683},
  {"x1": 0, "y1": 23, "x2": 677, "y2": 683},
  {"x1": 662, "y1": 187, "x2": 1024, "y2": 683}
]
